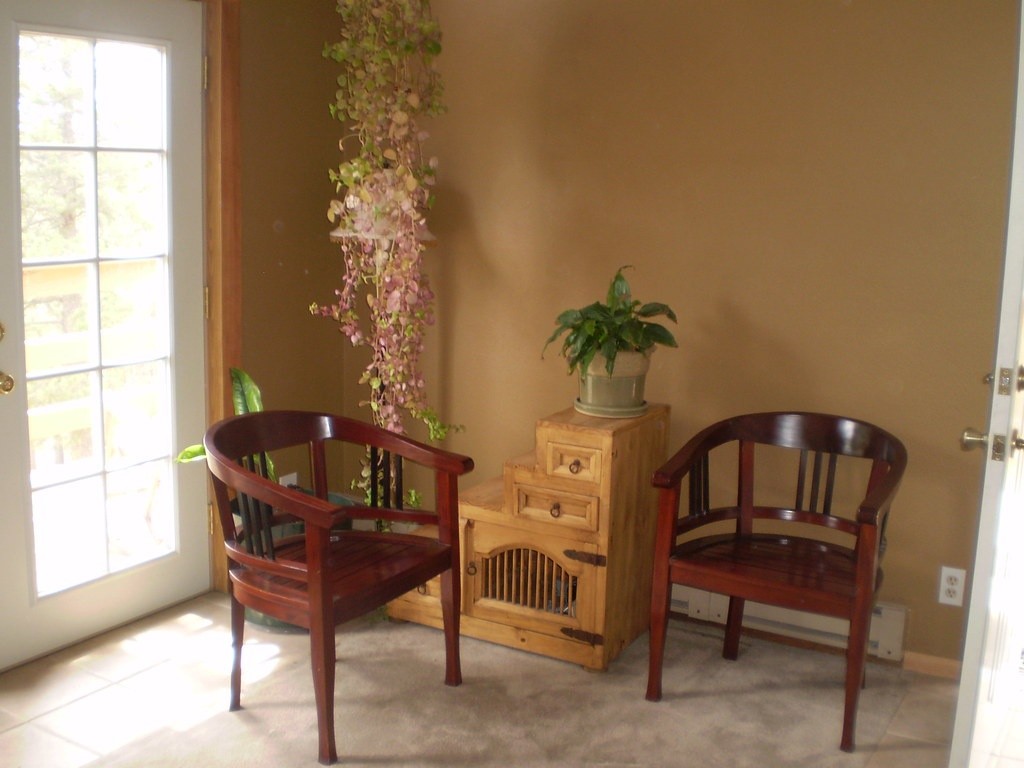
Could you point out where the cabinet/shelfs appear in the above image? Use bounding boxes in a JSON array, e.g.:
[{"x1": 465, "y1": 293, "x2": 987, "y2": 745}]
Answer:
[{"x1": 385, "y1": 403, "x2": 670, "y2": 673}]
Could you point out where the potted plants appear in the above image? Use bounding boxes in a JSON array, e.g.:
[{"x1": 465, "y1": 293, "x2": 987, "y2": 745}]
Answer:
[
  {"x1": 541, "y1": 265, "x2": 679, "y2": 418},
  {"x1": 177, "y1": 369, "x2": 355, "y2": 630}
]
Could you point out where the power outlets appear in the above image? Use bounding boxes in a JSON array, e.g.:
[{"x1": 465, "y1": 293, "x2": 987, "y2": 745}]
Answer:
[{"x1": 938, "y1": 565, "x2": 967, "y2": 606}]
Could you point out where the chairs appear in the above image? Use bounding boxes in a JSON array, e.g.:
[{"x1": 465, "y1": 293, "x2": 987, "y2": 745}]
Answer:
[
  {"x1": 205, "y1": 409, "x2": 474, "y2": 766},
  {"x1": 644, "y1": 412, "x2": 908, "y2": 753}
]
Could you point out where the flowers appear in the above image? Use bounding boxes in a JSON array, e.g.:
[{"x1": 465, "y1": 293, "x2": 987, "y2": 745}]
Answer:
[{"x1": 310, "y1": 0, "x2": 468, "y2": 535}]
